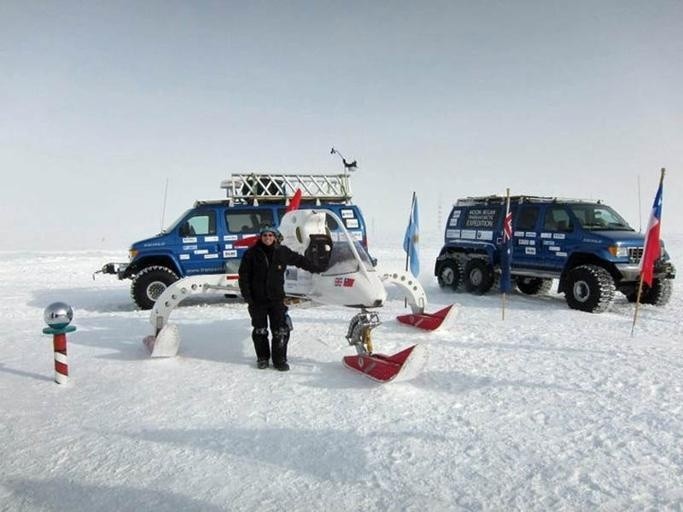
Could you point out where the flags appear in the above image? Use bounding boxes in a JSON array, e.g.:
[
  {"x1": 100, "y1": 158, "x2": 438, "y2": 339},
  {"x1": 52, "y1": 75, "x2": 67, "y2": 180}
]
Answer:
[
  {"x1": 638, "y1": 183, "x2": 663, "y2": 288},
  {"x1": 499, "y1": 195, "x2": 512, "y2": 296},
  {"x1": 403, "y1": 194, "x2": 420, "y2": 278}
]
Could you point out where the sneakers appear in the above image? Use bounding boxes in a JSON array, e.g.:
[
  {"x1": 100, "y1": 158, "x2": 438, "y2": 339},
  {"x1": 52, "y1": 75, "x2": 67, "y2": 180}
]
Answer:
[
  {"x1": 258, "y1": 360, "x2": 268, "y2": 369},
  {"x1": 273, "y1": 362, "x2": 289, "y2": 371}
]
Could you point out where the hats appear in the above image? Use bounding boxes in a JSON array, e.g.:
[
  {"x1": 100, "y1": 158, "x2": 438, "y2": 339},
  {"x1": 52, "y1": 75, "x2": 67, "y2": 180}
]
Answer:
[{"x1": 259, "y1": 225, "x2": 277, "y2": 233}]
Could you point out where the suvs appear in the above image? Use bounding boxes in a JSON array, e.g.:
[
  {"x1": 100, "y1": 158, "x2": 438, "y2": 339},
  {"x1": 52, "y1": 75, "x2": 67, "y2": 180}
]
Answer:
[
  {"x1": 117, "y1": 172, "x2": 377, "y2": 309},
  {"x1": 435, "y1": 196, "x2": 676, "y2": 312}
]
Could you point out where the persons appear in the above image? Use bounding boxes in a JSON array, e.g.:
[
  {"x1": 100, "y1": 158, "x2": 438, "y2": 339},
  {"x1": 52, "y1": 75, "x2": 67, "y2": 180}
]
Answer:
[{"x1": 238, "y1": 222, "x2": 328, "y2": 371}]
